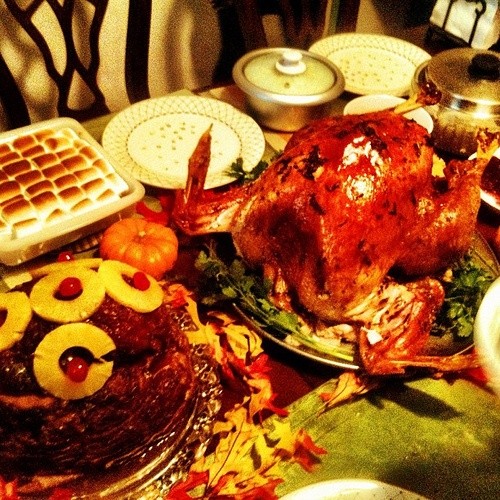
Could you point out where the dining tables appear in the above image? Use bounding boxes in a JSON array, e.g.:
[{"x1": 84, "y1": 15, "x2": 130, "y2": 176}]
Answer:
[{"x1": 0, "y1": 80, "x2": 499, "y2": 499}]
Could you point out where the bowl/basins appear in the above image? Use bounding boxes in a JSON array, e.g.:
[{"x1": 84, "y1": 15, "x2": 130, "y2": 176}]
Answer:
[{"x1": 344, "y1": 94, "x2": 433, "y2": 137}]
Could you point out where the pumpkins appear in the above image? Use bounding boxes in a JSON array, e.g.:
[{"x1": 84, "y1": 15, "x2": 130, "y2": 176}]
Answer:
[{"x1": 99, "y1": 218, "x2": 178, "y2": 280}]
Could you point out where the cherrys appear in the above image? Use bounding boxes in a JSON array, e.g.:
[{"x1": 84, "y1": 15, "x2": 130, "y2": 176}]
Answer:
[
  {"x1": 56, "y1": 253, "x2": 75, "y2": 263},
  {"x1": 132, "y1": 272, "x2": 150, "y2": 291},
  {"x1": 67, "y1": 358, "x2": 88, "y2": 383},
  {"x1": 58, "y1": 276, "x2": 81, "y2": 297}
]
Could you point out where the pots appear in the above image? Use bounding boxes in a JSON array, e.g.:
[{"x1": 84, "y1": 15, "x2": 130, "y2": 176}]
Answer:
[
  {"x1": 232, "y1": 47, "x2": 346, "y2": 134},
  {"x1": 407, "y1": 44, "x2": 500, "y2": 158}
]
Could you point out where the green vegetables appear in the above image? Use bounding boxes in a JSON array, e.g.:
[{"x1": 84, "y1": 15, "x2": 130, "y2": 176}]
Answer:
[{"x1": 196, "y1": 149, "x2": 495, "y2": 363}]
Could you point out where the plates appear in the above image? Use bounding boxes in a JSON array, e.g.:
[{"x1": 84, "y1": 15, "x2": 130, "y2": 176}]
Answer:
[
  {"x1": 190, "y1": 200, "x2": 500, "y2": 373},
  {"x1": 306, "y1": 31, "x2": 433, "y2": 99},
  {"x1": 101, "y1": 94, "x2": 265, "y2": 191},
  {"x1": 0, "y1": 390, "x2": 204, "y2": 499}
]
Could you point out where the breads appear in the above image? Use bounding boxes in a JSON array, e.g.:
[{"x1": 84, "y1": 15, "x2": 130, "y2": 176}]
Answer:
[{"x1": 0, "y1": 126, "x2": 129, "y2": 243}]
